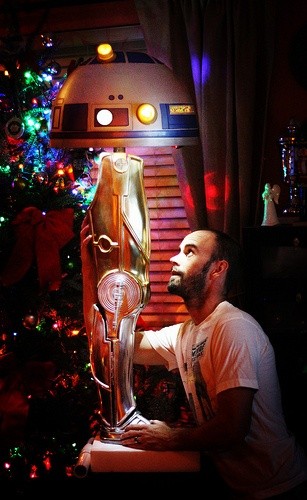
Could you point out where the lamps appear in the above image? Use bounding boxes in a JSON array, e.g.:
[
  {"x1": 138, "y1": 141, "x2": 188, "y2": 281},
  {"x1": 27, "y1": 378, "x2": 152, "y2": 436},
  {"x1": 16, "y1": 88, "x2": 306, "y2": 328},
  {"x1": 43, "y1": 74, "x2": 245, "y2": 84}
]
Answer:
[
  {"x1": 278, "y1": 112, "x2": 307, "y2": 218},
  {"x1": 136, "y1": 103, "x2": 157, "y2": 125},
  {"x1": 97, "y1": 43, "x2": 116, "y2": 62}
]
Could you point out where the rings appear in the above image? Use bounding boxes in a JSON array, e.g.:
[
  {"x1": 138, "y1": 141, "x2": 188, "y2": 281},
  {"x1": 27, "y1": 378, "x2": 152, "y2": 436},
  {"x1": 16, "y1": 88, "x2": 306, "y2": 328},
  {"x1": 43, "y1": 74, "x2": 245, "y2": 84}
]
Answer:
[{"x1": 133, "y1": 436, "x2": 139, "y2": 445}]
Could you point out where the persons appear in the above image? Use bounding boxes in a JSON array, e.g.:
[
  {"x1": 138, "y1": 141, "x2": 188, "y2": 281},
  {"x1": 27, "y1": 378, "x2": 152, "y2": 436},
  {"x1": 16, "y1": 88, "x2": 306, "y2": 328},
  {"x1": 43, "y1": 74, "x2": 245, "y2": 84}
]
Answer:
[{"x1": 79, "y1": 213, "x2": 307, "y2": 500}]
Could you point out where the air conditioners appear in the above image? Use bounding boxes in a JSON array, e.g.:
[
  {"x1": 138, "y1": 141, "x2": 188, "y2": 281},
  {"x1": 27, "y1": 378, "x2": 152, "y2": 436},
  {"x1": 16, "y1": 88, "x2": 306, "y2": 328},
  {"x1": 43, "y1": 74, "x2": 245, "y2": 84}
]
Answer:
[{"x1": 40, "y1": 24, "x2": 146, "y2": 60}]
